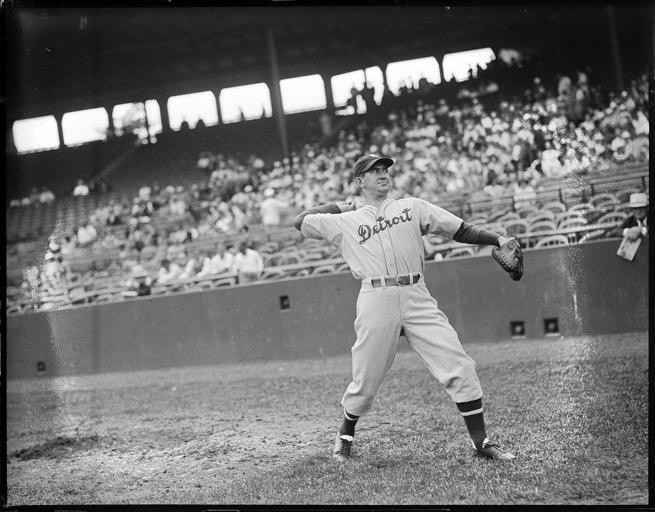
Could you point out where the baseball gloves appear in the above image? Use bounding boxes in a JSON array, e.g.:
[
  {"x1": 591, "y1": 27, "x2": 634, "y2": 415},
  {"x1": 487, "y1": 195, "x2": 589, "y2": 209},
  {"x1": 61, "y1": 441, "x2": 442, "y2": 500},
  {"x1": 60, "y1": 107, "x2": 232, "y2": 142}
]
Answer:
[{"x1": 492, "y1": 237, "x2": 524, "y2": 281}]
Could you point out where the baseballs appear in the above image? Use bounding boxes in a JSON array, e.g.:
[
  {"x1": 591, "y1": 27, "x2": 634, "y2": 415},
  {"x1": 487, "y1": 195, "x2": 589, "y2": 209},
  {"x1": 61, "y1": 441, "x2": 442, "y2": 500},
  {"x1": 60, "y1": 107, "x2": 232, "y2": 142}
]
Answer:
[{"x1": 353, "y1": 201, "x2": 362, "y2": 210}]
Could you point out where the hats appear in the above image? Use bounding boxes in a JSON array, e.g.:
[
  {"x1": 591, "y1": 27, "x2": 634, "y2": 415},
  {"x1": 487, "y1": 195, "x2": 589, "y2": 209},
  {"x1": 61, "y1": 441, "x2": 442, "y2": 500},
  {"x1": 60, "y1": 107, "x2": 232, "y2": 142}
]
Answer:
[{"x1": 352, "y1": 153, "x2": 393, "y2": 178}]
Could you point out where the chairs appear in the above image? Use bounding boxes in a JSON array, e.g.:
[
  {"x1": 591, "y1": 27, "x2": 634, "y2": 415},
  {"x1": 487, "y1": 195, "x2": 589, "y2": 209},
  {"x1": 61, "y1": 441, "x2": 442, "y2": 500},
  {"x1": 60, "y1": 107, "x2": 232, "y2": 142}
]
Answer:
[{"x1": 7, "y1": 189, "x2": 650, "y2": 315}]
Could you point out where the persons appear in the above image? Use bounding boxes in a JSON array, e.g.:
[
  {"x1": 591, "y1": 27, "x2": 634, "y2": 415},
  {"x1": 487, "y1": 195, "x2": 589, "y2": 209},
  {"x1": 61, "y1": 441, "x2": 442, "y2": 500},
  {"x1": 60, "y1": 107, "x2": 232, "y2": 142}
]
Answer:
[
  {"x1": 292, "y1": 152, "x2": 525, "y2": 464},
  {"x1": 6, "y1": 53, "x2": 650, "y2": 318}
]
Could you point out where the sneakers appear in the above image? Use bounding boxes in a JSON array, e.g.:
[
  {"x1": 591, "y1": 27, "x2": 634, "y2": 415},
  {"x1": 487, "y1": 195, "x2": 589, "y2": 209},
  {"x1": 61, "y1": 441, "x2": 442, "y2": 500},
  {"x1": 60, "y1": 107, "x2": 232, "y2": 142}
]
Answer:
[
  {"x1": 473, "y1": 440, "x2": 516, "y2": 463},
  {"x1": 333, "y1": 431, "x2": 355, "y2": 462}
]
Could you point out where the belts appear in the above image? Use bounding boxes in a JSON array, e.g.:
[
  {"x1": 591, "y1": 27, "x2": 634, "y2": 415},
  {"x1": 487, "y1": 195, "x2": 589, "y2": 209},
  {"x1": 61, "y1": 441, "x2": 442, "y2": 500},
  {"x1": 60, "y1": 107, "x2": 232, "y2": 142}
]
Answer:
[{"x1": 371, "y1": 276, "x2": 418, "y2": 287}]
